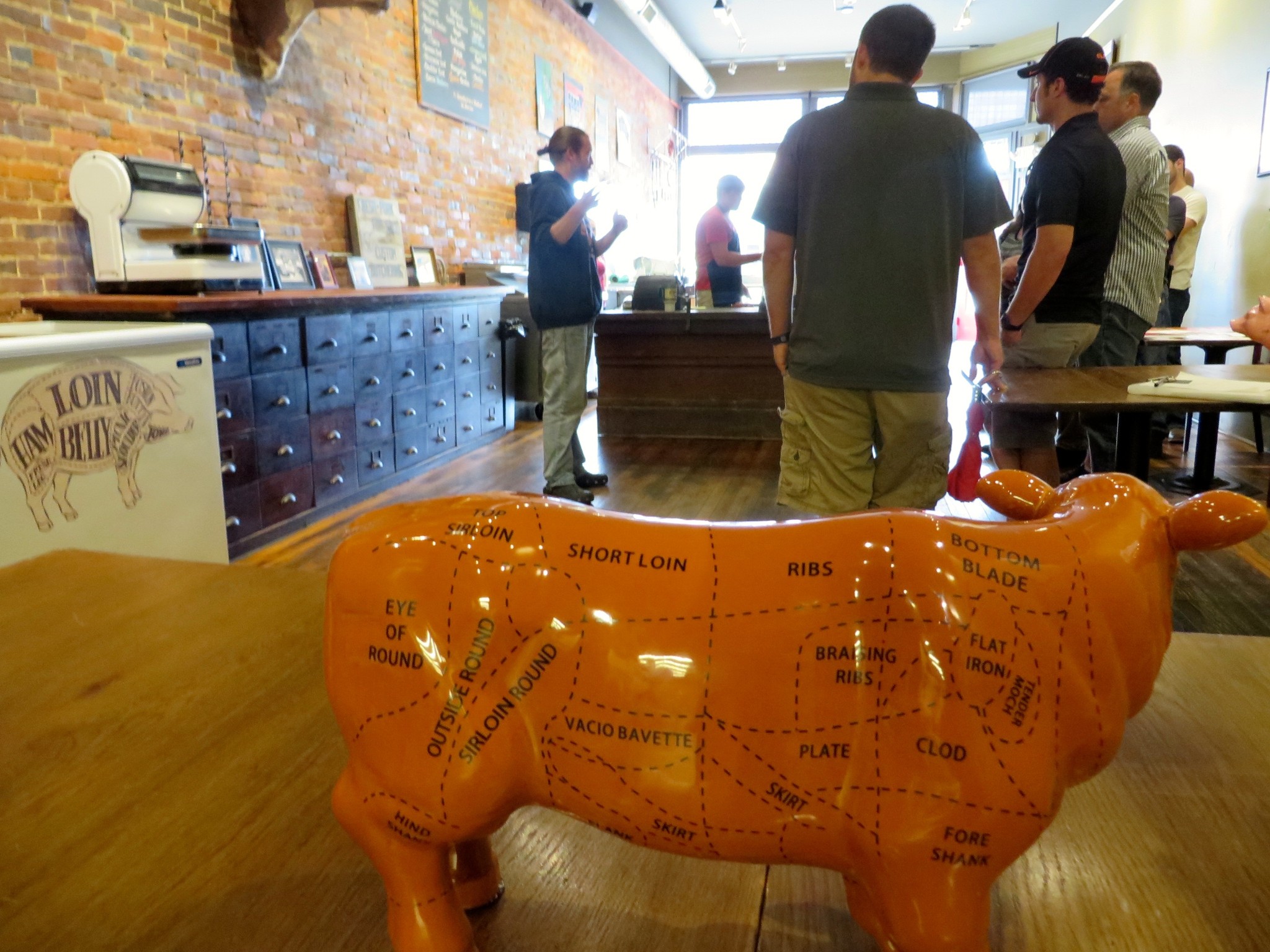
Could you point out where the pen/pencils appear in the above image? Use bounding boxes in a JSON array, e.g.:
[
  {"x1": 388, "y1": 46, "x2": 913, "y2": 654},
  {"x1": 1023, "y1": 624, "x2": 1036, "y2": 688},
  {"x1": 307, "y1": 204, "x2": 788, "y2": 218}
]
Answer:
[{"x1": 1154, "y1": 375, "x2": 1167, "y2": 388}]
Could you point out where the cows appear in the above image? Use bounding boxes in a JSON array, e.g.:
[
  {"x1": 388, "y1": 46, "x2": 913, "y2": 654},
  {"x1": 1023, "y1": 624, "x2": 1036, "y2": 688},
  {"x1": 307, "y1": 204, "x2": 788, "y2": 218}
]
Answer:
[{"x1": 323, "y1": 466, "x2": 1269, "y2": 952}]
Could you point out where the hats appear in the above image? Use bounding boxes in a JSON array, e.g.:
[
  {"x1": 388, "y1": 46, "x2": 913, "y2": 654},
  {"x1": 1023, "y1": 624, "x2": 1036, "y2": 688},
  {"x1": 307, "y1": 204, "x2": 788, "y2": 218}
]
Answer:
[{"x1": 1017, "y1": 37, "x2": 1108, "y2": 88}]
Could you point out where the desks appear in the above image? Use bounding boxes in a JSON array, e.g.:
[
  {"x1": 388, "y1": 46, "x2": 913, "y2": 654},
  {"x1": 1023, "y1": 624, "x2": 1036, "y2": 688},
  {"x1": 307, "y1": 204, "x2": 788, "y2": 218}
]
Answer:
[
  {"x1": 753, "y1": 626, "x2": 1270, "y2": 952},
  {"x1": 1137, "y1": 321, "x2": 1267, "y2": 494},
  {"x1": 958, "y1": 360, "x2": 1270, "y2": 506},
  {"x1": 0, "y1": 543, "x2": 772, "y2": 948}
]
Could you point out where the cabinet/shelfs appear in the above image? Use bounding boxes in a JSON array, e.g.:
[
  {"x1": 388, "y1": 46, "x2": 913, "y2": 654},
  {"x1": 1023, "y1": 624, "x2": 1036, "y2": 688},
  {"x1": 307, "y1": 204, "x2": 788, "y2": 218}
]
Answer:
[{"x1": 38, "y1": 288, "x2": 506, "y2": 549}]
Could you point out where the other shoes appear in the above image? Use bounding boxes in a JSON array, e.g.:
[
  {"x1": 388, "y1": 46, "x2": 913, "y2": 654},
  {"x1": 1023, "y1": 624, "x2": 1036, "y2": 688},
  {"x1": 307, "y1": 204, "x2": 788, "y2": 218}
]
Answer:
[
  {"x1": 1150, "y1": 441, "x2": 1165, "y2": 458},
  {"x1": 575, "y1": 472, "x2": 608, "y2": 488},
  {"x1": 543, "y1": 484, "x2": 595, "y2": 504},
  {"x1": 982, "y1": 444, "x2": 991, "y2": 456},
  {"x1": 1168, "y1": 424, "x2": 1186, "y2": 442},
  {"x1": 1056, "y1": 445, "x2": 1089, "y2": 476}
]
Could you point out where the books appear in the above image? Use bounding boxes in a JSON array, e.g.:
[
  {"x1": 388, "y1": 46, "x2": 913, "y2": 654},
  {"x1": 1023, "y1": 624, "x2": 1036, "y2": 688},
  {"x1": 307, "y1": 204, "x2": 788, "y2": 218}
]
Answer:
[{"x1": 346, "y1": 194, "x2": 409, "y2": 288}]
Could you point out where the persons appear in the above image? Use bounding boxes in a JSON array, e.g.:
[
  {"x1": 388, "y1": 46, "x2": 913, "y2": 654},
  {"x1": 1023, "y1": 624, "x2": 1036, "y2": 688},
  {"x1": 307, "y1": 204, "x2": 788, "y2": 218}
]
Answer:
[
  {"x1": 527, "y1": 125, "x2": 628, "y2": 505},
  {"x1": 994, "y1": 37, "x2": 1207, "y2": 486},
  {"x1": 751, "y1": 4, "x2": 1006, "y2": 521},
  {"x1": 694, "y1": 174, "x2": 765, "y2": 309}
]
